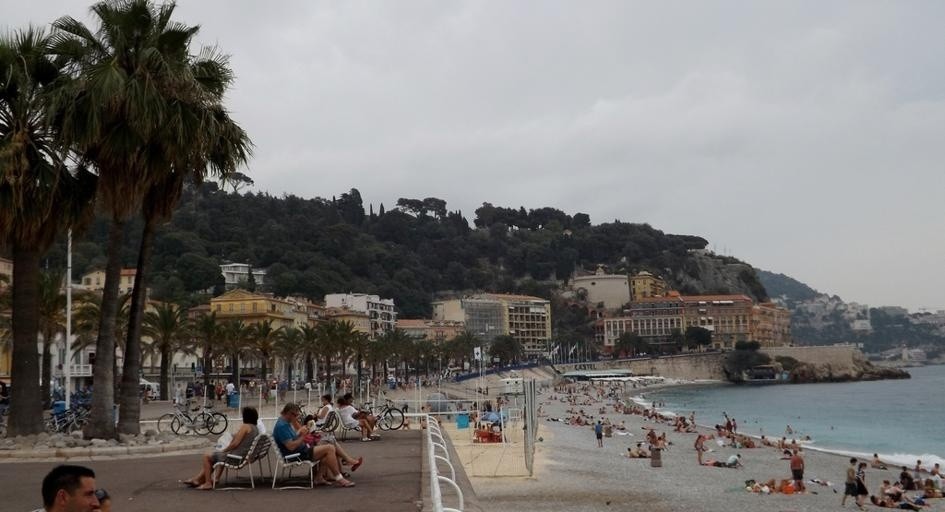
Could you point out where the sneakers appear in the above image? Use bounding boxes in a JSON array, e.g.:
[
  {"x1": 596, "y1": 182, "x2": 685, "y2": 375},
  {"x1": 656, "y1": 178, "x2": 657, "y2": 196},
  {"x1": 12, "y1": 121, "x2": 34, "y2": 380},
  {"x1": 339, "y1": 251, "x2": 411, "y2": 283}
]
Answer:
[
  {"x1": 370, "y1": 432, "x2": 380, "y2": 436},
  {"x1": 363, "y1": 438, "x2": 371, "y2": 442}
]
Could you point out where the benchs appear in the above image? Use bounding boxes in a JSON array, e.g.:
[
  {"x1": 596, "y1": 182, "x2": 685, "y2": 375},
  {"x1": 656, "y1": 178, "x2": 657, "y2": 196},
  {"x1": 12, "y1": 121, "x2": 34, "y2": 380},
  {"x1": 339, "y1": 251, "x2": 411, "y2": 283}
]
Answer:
[
  {"x1": 317, "y1": 411, "x2": 339, "y2": 435},
  {"x1": 336, "y1": 409, "x2": 363, "y2": 442},
  {"x1": 268, "y1": 415, "x2": 321, "y2": 489},
  {"x1": 212, "y1": 432, "x2": 272, "y2": 491}
]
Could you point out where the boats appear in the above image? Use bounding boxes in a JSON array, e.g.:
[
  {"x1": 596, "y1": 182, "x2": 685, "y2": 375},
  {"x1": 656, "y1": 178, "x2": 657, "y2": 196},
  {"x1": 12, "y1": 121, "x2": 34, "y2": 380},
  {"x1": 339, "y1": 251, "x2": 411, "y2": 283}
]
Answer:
[{"x1": 735, "y1": 363, "x2": 791, "y2": 384}]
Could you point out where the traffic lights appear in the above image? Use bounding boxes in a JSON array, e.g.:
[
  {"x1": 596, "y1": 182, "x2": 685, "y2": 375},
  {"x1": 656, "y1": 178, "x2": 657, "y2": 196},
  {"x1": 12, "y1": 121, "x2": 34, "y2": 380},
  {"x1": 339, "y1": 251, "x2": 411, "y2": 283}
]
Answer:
[{"x1": 190, "y1": 362, "x2": 195, "y2": 373}]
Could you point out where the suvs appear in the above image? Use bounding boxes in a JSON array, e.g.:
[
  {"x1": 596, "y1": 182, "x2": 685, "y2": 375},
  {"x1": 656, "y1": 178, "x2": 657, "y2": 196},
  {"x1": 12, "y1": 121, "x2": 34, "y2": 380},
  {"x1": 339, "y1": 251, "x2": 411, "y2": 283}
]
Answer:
[{"x1": 139, "y1": 383, "x2": 159, "y2": 400}]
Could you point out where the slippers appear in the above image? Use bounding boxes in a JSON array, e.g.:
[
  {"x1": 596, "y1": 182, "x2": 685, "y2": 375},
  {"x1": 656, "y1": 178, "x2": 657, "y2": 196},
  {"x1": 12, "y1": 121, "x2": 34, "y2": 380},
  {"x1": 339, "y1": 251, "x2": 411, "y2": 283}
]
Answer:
[
  {"x1": 314, "y1": 456, "x2": 363, "y2": 488},
  {"x1": 196, "y1": 483, "x2": 212, "y2": 489},
  {"x1": 183, "y1": 479, "x2": 200, "y2": 486}
]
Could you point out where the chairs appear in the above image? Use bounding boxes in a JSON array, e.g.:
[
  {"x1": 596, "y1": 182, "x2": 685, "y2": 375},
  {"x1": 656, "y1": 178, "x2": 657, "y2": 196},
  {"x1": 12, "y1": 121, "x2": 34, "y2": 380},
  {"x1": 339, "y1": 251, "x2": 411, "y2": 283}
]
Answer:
[{"x1": 474, "y1": 430, "x2": 501, "y2": 443}]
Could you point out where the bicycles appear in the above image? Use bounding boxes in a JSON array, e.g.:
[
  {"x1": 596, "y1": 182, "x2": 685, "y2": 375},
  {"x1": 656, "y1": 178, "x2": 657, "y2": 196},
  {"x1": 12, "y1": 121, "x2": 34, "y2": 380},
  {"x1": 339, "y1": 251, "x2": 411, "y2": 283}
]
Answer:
[
  {"x1": 157, "y1": 400, "x2": 215, "y2": 436},
  {"x1": 170, "y1": 399, "x2": 228, "y2": 436},
  {"x1": 367, "y1": 406, "x2": 392, "y2": 432},
  {"x1": 354, "y1": 399, "x2": 404, "y2": 432},
  {"x1": 0, "y1": 382, "x2": 93, "y2": 439}
]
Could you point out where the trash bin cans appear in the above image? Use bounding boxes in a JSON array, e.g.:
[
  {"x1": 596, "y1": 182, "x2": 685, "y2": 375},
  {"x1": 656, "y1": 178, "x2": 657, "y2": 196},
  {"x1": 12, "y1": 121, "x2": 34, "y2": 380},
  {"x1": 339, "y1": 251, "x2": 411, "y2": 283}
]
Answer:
[
  {"x1": 53, "y1": 400, "x2": 65, "y2": 424},
  {"x1": 229, "y1": 391, "x2": 240, "y2": 408}
]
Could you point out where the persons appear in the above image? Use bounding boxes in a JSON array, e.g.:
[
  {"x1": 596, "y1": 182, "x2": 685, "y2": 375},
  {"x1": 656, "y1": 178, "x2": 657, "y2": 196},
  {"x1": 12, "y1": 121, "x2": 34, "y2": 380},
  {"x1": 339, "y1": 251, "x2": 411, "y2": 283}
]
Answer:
[
  {"x1": 29, "y1": 465, "x2": 100, "y2": 512},
  {"x1": 93, "y1": 488, "x2": 112, "y2": 512},
  {"x1": 144, "y1": 374, "x2": 523, "y2": 491},
  {"x1": 536, "y1": 371, "x2": 944, "y2": 508}
]
